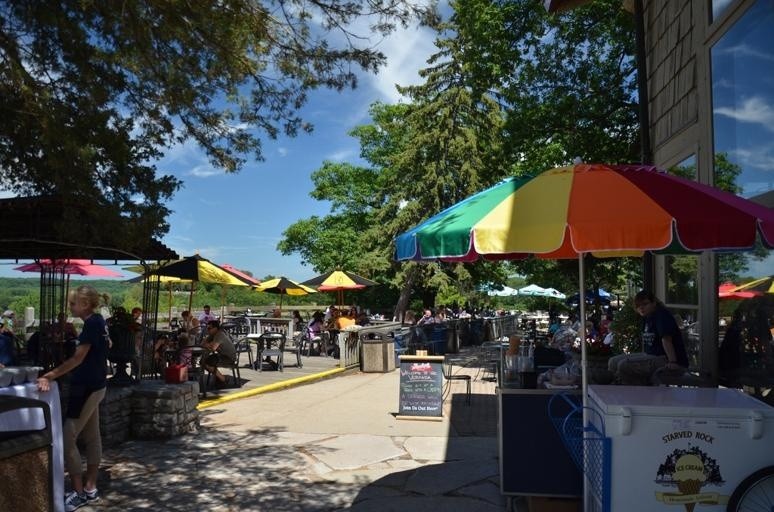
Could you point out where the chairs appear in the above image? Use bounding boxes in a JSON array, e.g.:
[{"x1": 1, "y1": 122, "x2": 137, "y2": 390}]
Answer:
[
  {"x1": 474, "y1": 307, "x2": 772, "y2": 405},
  {"x1": 442, "y1": 366, "x2": 474, "y2": 407},
  {"x1": 728, "y1": 463, "x2": 773, "y2": 512}
]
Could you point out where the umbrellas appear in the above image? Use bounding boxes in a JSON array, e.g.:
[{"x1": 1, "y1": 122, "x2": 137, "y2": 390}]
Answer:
[
  {"x1": 13, "y1": 257, "x2": 123, "y2": 318},
  {"x1": 717, "y1": 278, "x2": 763, "y2": 301},
  {"x1": 535, "y1": 285, "x2": 566, "y2": 313},
  {"x1": 0, "y1": 187, "x2": 179, "y2": 329},
  {"x1": 210, "y1": 260, "x2": 261, "y2": 329},
  {"x1": 395, "y1": 154, "x2": 773, "y2": 511},
  {"x1": 238, "y1": 271, "x2": 320, "y2": 316},
  {"x1": 517, "y1": 281, "x2": 547, "y2": 313},
  {"x1": 482, "y1": 280, "x2": 517, "y2": 300},
  {"x1": 297, "y1": 265, "x2": 381, "y2": 320},
  {"x1": 562, "y1": 288, "x2": 617, "y2": 306},
  {"x1": 118, "y1": 249, "x2": 263, "y2": 317},
  {"x1": 728, "y1": 271, "x2": 773, "y2": 293},
  {"x1": 118, "y1": 257, "x2": 194, "y2": 330}
]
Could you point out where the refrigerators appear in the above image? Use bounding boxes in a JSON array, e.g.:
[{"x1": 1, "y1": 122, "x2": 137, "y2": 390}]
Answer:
[{"x1": 587, "y1": 384, "x2": 774, "y2": 512}]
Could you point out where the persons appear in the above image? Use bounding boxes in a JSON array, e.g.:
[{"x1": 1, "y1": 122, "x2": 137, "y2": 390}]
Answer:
[
  {"x1": 34, "y1": 282, "x2": 109, "y2": 512},
  {"x1": 0, "y1": 318, "x2": 19, "y2": 368},
  {"x1": 505, "y1": 301, "x2": 617, "y2": 386},
  {"x1": 403, "y1": 304, "x2": 505, "y2": 342},
  {"x1": 607, "y1": 288, "x2": 689, "y2": 385},
  {"x1": 34, "y1": 303, "x2": 238, "y2": 394},
  {"x1": 293, "y1": 305, "x2": 372, "y2": 356}
]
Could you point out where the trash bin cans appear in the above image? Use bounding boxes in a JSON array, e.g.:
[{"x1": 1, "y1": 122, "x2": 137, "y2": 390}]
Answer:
[
  {"x1": 361, "y1": 331, "x2": 396, "y2": 373},
  {"x1": 0, "y1": 394, "x2": 54, "y2": 512}
]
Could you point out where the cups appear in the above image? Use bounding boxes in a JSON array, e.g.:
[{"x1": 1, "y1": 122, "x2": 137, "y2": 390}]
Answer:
[{"x1": 506, "y1": 353, "x2": 529, "y2": 371}]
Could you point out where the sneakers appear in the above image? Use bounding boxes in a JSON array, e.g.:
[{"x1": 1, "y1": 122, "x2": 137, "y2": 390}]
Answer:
[
  {"x1": 64, "y1": 489, "x2": 89, "y2": 511},
  {"x1": 83, "y1": 487, "x2": 100, "y2": 502},
  {"x1": 222, "y1": 375, "x2": 231, "y2": 387}
]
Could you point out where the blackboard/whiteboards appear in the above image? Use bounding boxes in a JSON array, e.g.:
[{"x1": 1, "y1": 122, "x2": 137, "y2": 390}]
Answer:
[{"x1": 395, "y1": 355, "x2": 445, "y2": 421}]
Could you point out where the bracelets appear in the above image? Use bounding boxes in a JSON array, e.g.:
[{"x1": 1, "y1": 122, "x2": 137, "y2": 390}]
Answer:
[{"x1": 669, "y1": 360, "x2": 678, "y2": 364}]
